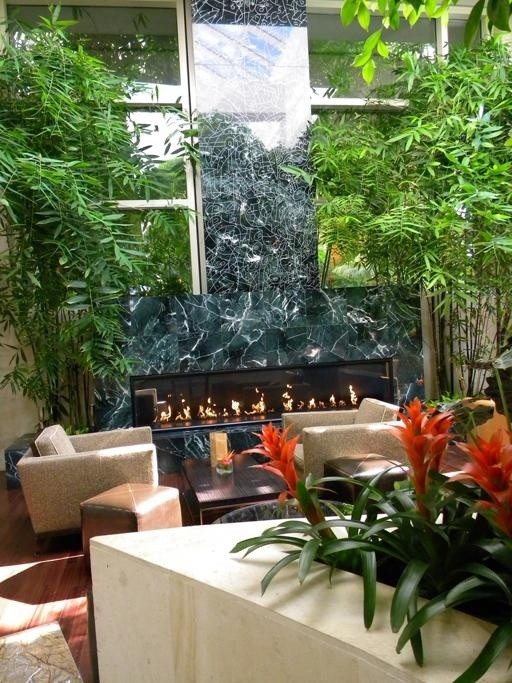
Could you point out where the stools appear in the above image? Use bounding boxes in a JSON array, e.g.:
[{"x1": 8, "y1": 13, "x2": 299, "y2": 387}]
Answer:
[
  {"x1": 76, "y1": 481, "x2": 183, "y2": 556},
  {"x1": 321, "y1": 453, "x2": 409, "y2": 505}
]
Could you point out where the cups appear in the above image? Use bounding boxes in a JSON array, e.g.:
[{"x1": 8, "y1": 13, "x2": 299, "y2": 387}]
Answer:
[{"x1": 215, "y1": 458, "x2": 234, "y2": 473}]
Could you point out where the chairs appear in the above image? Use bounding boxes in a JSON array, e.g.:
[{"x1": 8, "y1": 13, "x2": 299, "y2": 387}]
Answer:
[
  {"x1": 16, "y1": 423, "x2": 162, "y2": 547},
  {"x1": 278, "y1": 397, "x2": 416, "y2": 499}
]
